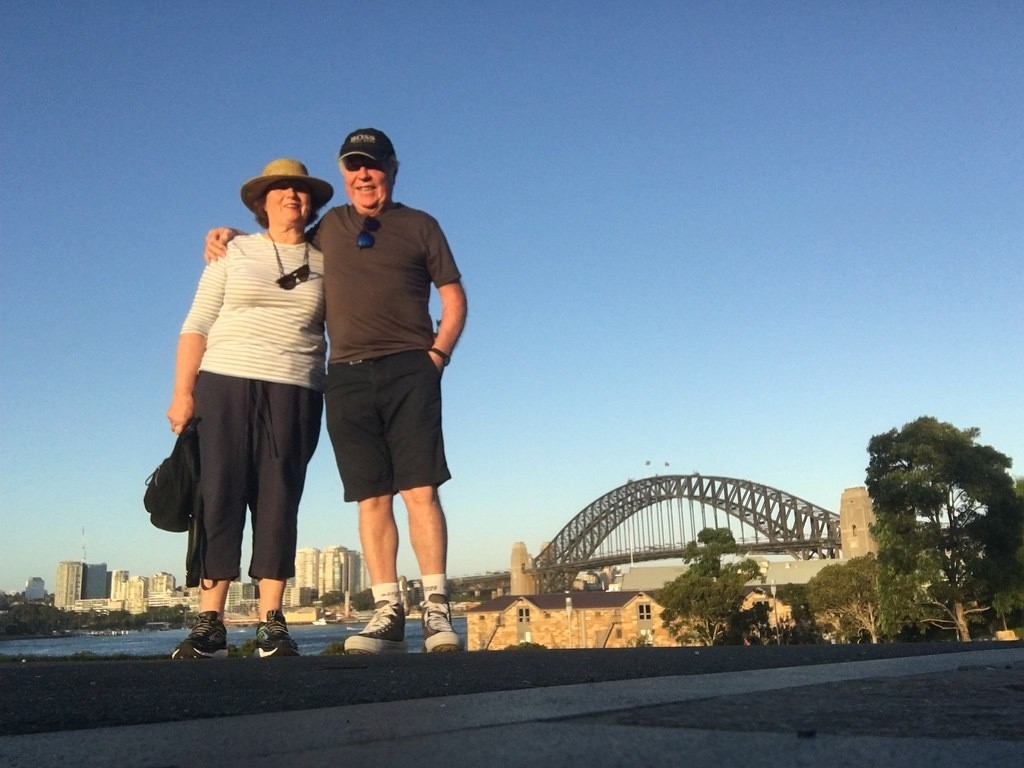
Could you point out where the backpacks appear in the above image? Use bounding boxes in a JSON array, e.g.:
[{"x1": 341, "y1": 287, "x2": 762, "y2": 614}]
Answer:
[{"x1": 144, "y1": 417, "x2": 204, "y2": 589}]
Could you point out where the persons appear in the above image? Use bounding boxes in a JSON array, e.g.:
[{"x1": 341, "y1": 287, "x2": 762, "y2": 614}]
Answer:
[
  {"x1": 205, "y1": 127, "x2": 468, "y2": 653},
  {"x1": 166, "y1": 159, "x2": 327, "y2": 659}
]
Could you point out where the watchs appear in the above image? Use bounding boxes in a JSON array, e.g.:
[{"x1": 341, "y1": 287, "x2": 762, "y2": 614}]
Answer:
[{"x1": 432, "y1": 348, "x2": 450, "y2": 366}]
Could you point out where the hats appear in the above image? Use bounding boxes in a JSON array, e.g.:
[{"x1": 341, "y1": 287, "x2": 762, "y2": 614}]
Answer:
[
  {"x1": 338, "y1": 128, "x2": 396, "y2": 161},
  {"x1": 240, "y1": 158, "x2": 334, "y2": 214}
]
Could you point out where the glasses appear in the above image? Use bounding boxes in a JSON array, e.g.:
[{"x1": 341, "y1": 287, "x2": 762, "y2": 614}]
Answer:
[
  {"x1": 357, "y1": 215, "x2": 381, "y2": 251},
  {"x1": 276, "y1": 250, "x2": 310, "y2": 290}
]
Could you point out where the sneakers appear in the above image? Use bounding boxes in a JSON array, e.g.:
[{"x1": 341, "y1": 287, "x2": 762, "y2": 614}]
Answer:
[
  {"x1": 170, "y1": 611, "x2": 228, "y2": 659},
  {"x1": 419, "y1": 593, "x2": 466, "y2": 653},
  {"x1": 344, "y1": 601, "x2": 408, "y2": 654},
  {"x1": 253, "y1": 610, "x2": 299, "y2": 658}
]
